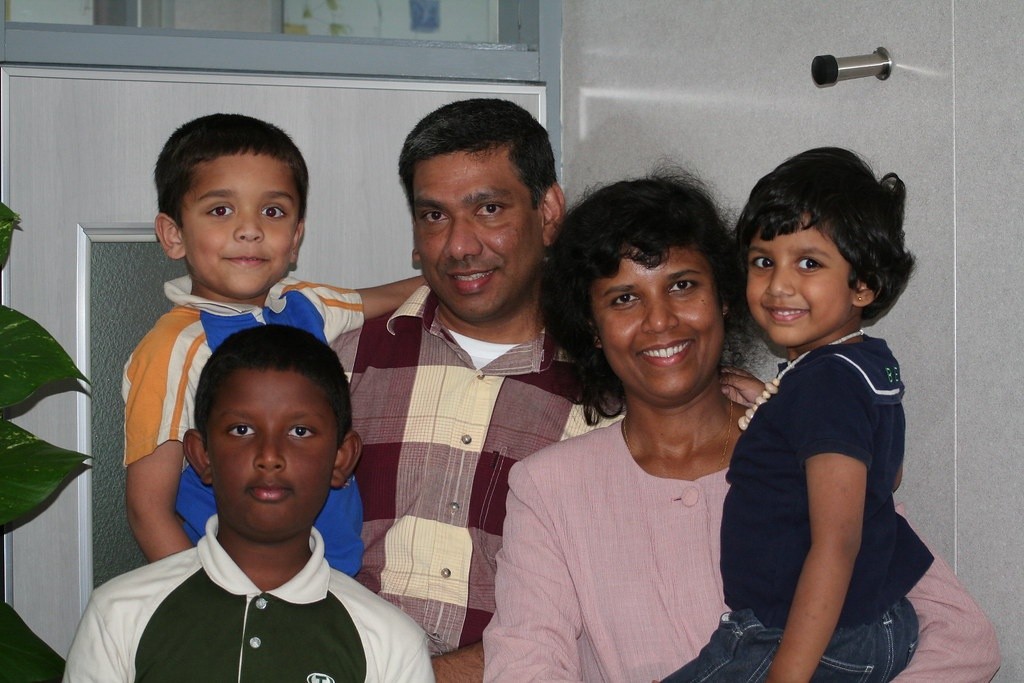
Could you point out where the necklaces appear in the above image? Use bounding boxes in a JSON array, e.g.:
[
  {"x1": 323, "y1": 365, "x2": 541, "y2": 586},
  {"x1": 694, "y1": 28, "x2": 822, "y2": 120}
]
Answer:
[
  {"x1": 737, "y1": 329, "x2": 866, "y2": 430},
  {"x1": 719, "y1": 400, "x2": 734, "y2": 471}
]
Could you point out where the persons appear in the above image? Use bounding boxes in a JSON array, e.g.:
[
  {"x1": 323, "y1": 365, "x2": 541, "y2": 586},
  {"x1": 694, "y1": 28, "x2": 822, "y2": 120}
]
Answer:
[
  {"x1": 326, "y1": 94, "x2": 628, "y2": 683},
  {"x1": 118, "y1": 111, "x2": 429, "y2": 577},
  {"x1": 477, "y1": 173, "x2": 1003, "y2": 683},
  {"x1": 662, "y1": 144, "x2": 937, "y2": 683},
  {"x1": 61, "y1": 323, "x2": 437, "y2": 683}
]
